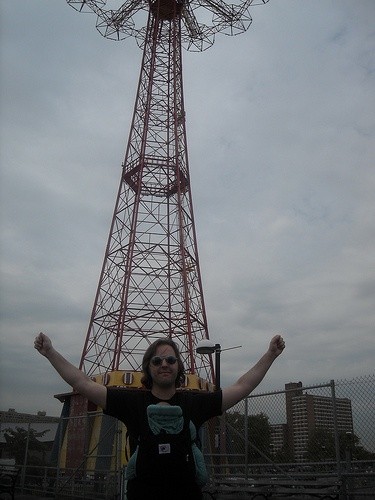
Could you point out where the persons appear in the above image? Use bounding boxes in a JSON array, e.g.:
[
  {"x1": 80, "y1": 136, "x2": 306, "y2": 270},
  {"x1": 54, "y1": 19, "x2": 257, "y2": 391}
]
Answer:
[{"x1": 34, "y1": 331, "x2": 286, "y2": 500}]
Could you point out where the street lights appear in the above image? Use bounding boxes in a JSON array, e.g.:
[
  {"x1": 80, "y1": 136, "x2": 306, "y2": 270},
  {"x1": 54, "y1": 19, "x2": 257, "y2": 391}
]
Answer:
[{"x1": 196, "y1": 339, "x2": 221, "y2": 483}]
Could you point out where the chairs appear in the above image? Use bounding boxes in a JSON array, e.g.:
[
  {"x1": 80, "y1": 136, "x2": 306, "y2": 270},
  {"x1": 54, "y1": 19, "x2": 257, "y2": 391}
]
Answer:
[{"x1": 0, "y1": 466, "x2": 23, "y2": 500}]
